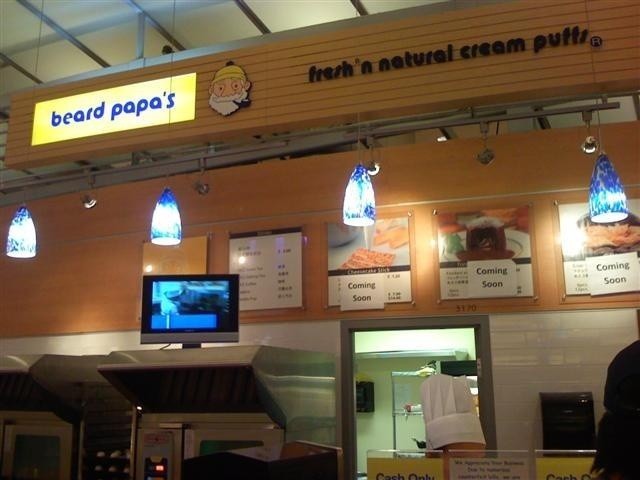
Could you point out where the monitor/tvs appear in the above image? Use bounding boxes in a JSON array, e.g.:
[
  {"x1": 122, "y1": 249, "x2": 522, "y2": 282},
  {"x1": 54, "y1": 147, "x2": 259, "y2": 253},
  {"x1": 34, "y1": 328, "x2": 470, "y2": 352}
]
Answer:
[{"x1": 140, "y1": 274, "x2": 239, "y2": 344}]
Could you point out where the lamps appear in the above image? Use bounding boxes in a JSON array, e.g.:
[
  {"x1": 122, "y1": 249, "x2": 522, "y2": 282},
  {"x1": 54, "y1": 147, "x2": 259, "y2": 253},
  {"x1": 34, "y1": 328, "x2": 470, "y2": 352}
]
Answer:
[
  {"x1": 5, "y1": 0, "x2": 43, "y2": 259},
  {"x1": 343, "y1": 0, "x2": 376, "y2": 226},
  {"x1": 150, "y1": 1, "x2": 182, "y2": 245},
  {"x1": 342, "y1": 103, "x2": 620, "y2": 176},
  {"x1": 585, "y1": 0, "x2": 630, "y2": 224},
  {"x1": 0, "y1": 141, "x2": 287, "y2": 208}
]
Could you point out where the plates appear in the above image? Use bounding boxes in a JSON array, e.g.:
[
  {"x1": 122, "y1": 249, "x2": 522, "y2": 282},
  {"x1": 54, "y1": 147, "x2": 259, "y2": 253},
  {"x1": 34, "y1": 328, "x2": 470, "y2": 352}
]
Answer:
[{"x1": 439, "y1": 227, "x2": 531, "y2": 264}]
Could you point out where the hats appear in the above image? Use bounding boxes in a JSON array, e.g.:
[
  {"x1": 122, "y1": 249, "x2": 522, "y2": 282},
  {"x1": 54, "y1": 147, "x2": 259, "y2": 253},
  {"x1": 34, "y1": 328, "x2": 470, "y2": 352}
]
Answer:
[{"x1": 420, "y1": 374, "x2": 487, "y2": 450}]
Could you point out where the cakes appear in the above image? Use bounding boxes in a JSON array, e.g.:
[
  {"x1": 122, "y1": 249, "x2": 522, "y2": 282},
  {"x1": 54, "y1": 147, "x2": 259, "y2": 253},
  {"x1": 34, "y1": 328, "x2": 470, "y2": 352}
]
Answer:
[{"x1": 466, "y1": 216, "x2": 506, "y2": 251}]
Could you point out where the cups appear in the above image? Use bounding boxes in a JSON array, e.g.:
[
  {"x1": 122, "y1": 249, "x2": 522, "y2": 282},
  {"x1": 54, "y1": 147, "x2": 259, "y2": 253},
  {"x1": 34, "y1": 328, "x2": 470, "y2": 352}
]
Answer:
[{"x1": 454, "y1": 350, "x2": 470, "y2": 362}]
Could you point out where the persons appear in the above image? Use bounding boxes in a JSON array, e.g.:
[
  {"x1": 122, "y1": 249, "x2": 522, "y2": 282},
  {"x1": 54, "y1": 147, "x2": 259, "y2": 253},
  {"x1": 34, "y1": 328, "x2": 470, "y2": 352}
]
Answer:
[
  {"x1": 421, "y1": 429, "x2": 487, "y2": 458},
  {"x1": 592, "y1": 413, "x2": 640, "y2": 479}
]
